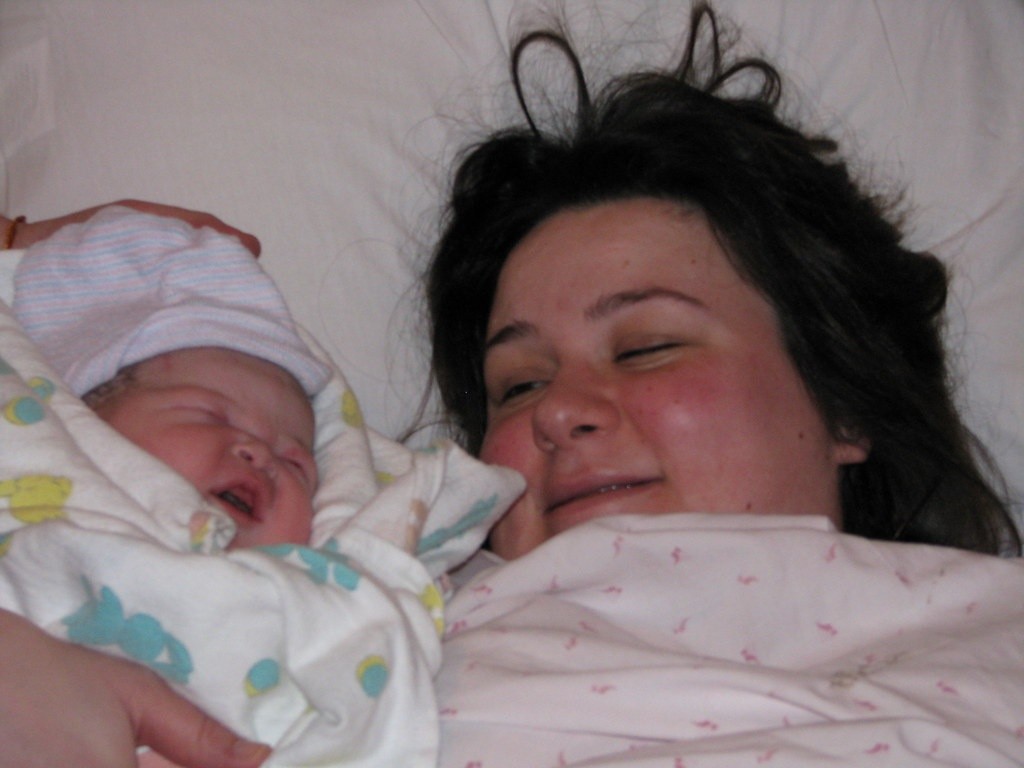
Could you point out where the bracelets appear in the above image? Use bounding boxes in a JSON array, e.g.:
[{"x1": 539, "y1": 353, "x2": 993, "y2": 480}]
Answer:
[{"x1": 7, "y1": 216, "x2": 25, "y2": 249}]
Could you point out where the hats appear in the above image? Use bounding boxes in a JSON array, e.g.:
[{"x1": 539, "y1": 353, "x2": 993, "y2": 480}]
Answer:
[{"x1": 10, "y1": 204, "x2": 333, "y2": 399}]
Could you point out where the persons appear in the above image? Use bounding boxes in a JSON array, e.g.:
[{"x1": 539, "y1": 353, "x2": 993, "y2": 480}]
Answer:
[
  {"x1": 427, "y1": 0, "x2": 1024, "y2": 768},
  {"x1": 0, "y1": 289, "x2": 321, "y2": 768},
  {"x1": 0, "y1": 198, "x2": 273, "y2": 768}
]
